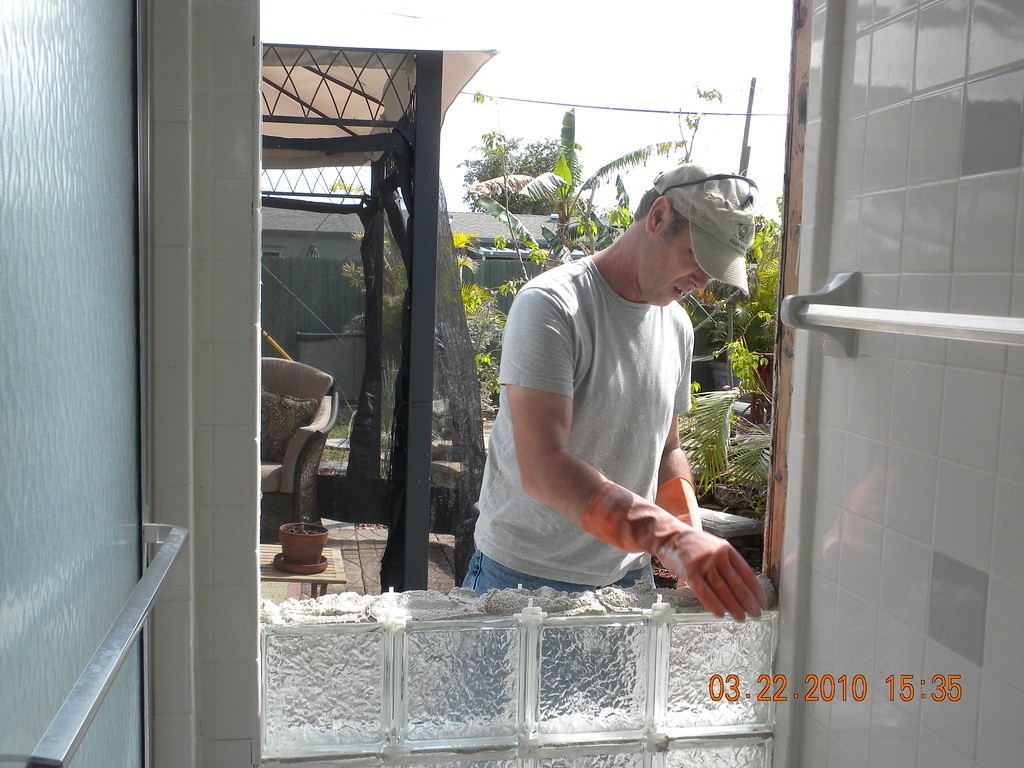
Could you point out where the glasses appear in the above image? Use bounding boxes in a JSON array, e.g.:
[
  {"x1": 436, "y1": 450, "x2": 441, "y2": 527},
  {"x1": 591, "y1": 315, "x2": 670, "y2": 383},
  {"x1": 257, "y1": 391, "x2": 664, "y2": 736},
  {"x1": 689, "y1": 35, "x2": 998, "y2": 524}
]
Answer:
[{"x1": 661, "y1": 174, "x2": 758, "y2": 210}]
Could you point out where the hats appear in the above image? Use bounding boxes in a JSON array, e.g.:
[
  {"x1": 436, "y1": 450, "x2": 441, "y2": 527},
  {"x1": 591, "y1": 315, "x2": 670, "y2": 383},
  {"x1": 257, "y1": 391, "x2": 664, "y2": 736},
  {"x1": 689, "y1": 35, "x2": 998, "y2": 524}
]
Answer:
[{"x1": 653, "y1": 164, "x2": 758, "y2": 295}]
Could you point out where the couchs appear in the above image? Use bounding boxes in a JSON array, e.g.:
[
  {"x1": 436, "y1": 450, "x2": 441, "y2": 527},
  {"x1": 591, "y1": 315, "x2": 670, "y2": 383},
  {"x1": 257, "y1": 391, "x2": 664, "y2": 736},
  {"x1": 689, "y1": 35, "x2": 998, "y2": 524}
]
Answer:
[{"x1": 261, "y1": 353, "x2": 339, "y2": 534}]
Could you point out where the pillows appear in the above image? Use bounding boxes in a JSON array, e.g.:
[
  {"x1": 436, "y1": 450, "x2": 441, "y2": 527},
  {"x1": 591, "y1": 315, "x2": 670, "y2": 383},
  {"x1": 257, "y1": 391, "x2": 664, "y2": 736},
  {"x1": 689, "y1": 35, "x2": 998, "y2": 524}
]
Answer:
[{"x1": 261, "y1": 385, "x2": 318, "y2": 464}]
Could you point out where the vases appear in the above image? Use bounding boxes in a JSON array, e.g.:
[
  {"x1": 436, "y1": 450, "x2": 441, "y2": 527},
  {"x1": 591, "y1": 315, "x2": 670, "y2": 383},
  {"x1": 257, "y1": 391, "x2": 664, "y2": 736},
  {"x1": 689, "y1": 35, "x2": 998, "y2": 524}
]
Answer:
[{"x1": 274, "y1": 523, "x2": 328, "y2": 574}]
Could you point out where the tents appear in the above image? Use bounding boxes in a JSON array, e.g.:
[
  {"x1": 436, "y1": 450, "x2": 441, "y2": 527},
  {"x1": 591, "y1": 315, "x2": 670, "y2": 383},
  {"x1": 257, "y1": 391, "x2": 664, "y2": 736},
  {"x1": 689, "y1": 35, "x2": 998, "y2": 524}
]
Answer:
[{"x1": 249, "y1": 2, "x2": 498, "y2": 596}]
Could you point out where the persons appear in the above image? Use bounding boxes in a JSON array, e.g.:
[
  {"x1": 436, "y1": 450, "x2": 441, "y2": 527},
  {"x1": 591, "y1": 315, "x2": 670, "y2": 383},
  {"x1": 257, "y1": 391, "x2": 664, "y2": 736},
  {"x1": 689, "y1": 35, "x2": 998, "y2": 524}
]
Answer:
[{"x1": 461, "y1": 163, "x2": 771, "y2": 624}]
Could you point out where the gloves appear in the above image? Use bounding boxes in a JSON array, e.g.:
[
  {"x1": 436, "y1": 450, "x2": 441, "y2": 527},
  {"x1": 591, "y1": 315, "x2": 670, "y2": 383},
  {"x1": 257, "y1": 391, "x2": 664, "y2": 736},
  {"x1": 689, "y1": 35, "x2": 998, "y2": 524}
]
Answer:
[
  {"x1": 577, "y1": 476, "x2": 771, "y2": 622},
  {"x1": 645, "y1": 477, "x2": 705, "y2": 590}
]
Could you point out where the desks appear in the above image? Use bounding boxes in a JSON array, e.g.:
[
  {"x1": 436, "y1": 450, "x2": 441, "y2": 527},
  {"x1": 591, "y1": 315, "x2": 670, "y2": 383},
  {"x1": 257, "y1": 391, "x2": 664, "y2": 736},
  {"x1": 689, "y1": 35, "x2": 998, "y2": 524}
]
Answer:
[{"x1": 260, "y1": 543, "x2": 347, "y2": 600}]
